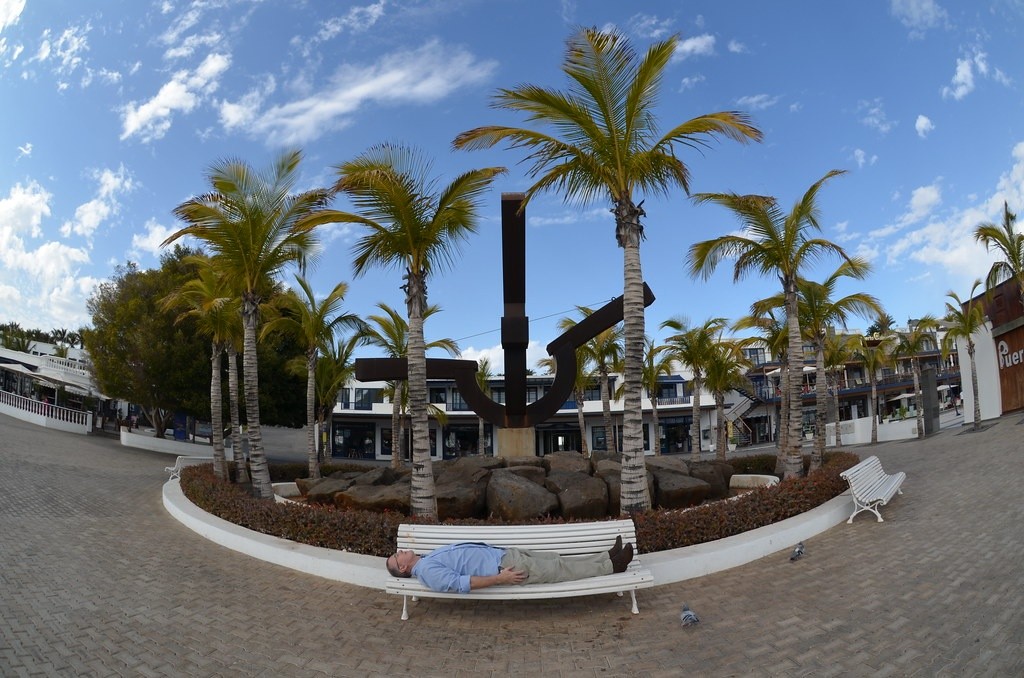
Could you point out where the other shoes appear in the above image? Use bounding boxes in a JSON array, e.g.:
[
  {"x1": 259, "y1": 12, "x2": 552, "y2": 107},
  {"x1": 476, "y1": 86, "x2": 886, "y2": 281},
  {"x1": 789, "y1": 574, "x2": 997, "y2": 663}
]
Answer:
[
  {"x1": 608, "y1": 534, "x2": 622, "y2": 559},
  {"x1": 610, "y1": 542, "x2": 633, "y2": 574}
]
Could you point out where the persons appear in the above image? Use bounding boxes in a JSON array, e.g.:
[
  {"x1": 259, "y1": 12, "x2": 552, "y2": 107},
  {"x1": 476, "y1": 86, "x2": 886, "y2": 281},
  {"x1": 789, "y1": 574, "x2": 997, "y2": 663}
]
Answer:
[
  {"x1": 386, "y1": 535, "x2": 633, "y2": 594},
  {"x1": 11, "y1": 389, "x2": 15, "y2": 394},
  {"x1": 41, "y1": 397, "x2": 48, "y2": 415}
]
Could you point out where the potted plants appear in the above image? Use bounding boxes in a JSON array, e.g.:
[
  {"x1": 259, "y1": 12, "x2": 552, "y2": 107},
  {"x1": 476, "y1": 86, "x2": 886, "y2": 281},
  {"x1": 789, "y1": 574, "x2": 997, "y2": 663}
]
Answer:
[
  {"x1": 882, "y1": 415, "x2": 890, "y2": 424},
  {"x1": 727, "y1": 437, "x2": 737, "y2": 452}
]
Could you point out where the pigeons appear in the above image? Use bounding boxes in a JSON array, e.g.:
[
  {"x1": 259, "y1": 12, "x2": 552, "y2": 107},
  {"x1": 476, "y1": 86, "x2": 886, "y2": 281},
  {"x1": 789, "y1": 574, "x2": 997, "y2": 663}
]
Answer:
[
  {"x1": 789, "y1": 540, "x2": 805, "y2": 562},
  {"x1": 679, "y1": 601, "x2": 700, "y2": 629}
]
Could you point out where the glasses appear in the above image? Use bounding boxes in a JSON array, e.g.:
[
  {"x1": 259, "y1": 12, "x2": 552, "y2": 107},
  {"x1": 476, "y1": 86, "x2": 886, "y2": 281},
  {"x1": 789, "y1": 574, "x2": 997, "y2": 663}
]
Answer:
[{"x1": 394, "y1": 553, "x2": 400, "y2": 570}]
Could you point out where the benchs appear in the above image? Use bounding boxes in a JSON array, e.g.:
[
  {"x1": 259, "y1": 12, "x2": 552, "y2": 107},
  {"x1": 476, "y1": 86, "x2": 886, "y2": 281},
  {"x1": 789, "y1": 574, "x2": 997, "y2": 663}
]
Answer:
[
  {"x1": 839, "y1": 455, "x2": 907, "y2": 525},
  {"x1": 164, "y1": 456, "x2": 214, "y2": 480},
  {"x1": 387, "y1": 521, "x2": 651, "y2": 621}
]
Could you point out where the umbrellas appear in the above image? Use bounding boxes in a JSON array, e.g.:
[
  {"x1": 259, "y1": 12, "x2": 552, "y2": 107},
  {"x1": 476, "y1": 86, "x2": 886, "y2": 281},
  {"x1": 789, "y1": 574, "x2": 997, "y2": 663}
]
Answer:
[
  {"x1": 32, "y1": 370, "x2": 86, "y2": 407},
  {"x1": 765, "y1": 363, "x2": 845, "y2": 389},
  {"x1": 888, "y1": 385, "x2": 959, "y2": 411},
  {"x1": 0, "y1": 363, "x2": 33, "y2": 395}
]
